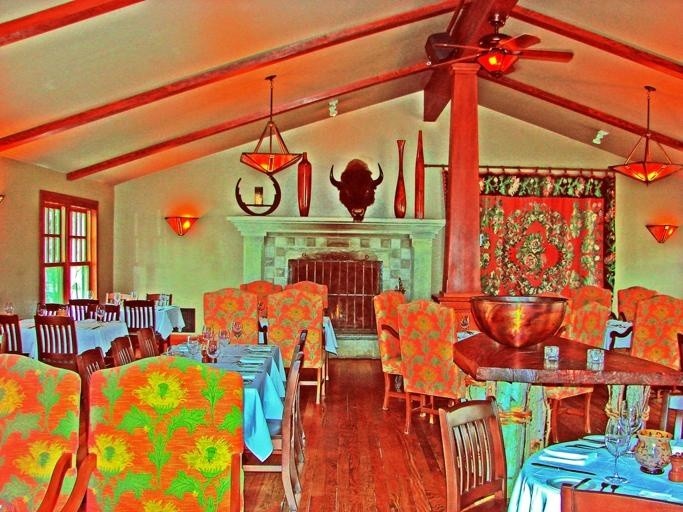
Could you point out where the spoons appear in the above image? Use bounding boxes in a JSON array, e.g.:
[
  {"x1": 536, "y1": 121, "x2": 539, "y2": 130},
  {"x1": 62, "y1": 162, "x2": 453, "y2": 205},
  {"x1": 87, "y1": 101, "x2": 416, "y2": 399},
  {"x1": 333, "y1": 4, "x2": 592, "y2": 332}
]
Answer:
[
  {"x1": 599, "y1": 482, "x2": 610, "y2": 493},
  {"x1": 611, "y1": 485, "x2": 619, "y2": 493}
]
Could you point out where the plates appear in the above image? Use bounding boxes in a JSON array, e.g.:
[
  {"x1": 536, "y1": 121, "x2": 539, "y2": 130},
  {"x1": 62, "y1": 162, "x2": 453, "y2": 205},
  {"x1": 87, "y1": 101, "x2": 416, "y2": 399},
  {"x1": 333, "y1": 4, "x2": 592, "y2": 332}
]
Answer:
[{"x1": 547, "y1": 477, "x2": 589, "y2": 491}]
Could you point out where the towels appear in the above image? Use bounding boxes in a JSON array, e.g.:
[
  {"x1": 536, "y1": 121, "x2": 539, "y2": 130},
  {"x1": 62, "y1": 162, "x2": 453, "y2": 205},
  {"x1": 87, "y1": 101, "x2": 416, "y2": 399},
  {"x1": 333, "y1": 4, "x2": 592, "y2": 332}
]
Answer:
[
  {"x1": 249, "y1": 345, "x2": 272, "y2": 351},
  {"x1": 241, "y1": 374, "x2": 254, "y2": 380},
  {"x1": 544, "y1": 446, "x2": 597, "y2": 459},
  {"x1": 540, "y1": 454, "x2": 595, "y2": 466},
  {"x1": 240, "y1": 357, "x2": 266, "y2": 364}
]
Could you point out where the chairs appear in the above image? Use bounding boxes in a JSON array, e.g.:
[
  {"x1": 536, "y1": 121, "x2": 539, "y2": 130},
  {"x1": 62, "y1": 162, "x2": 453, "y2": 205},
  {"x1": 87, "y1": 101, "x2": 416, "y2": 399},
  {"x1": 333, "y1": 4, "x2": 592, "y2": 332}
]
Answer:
[
  {"x1": 146, "y1": 293, "x2": 172, "y2": 305},
  {"x1": 38, "y1": 303, "x2": 65, "y2": 319},
  {"x1": 629, "y1": 296, "x2": 683, "y2": 398},
  {"x1": 97, "y1": 304, "x2": 120, "y2": 322},
  {"x1": 374, "y1": 291, "x2": 426, "y2": 418},
  {"x1": 138, "y1": 328, "x2": 159, "y2": 358},
  {"x1": 267, "y1": 289, "x2": 325, "y2": 405},
  {"x1": 289, "y1": 329, "x2": 306, "y2": 462},
  {"x1": 34, "y1": 315, "x2": 78, "y2": 374},
  {"x1": 0, "y1": 315, "x2": 29, "y2": 356},
  {"x1": 203, "y1": 289, "x2": 267, "y2": 348},
  {"x1": 60, "y1": 355, "x2": 243, "y2": 511},
  {"x1": 0, "y1": 354, "x2": 82, "y2": 512},
  {"x1": 438, "y1": 396, "x2": 510, "y2": 512},
  {"x1": 561, "y1": 485, "x2": 683, "y2": 512},
  {"x1": 617, "y1": 287, "x2": 659, "y2": 323},
  {"x1": 241, "y1": 282, "x2": 282, "y2": 318},
  {"x1": 68, "y1": 299, "x2": 99, "y2": 322},
  {"x1": 242, "y1": 351, "x2": 304, "y2": 512},
  {"x1": 546, "y1": 286, "x2": 612, "y2": 444},
  {"x1": 285, "y1": 281, "x2": 330, "y2": 381},
  {"x1": 111, "y1": 336, "x2": 135, "y2": 367},
  {"x1": 77, "y1": 347, "x2": 106, "y2": 384},
  {"x1": 123, "y1": 299, "x2": 160, "y2": 357},
  {"x1": 659, "y1": 394, "x2": 683, "y2": 442},
  {"x1": 106, "y1": 294, "x2": 131, "y2": 304},
  {"x1": 397, "y1": 300, "x2": 467, "y2": 434}
]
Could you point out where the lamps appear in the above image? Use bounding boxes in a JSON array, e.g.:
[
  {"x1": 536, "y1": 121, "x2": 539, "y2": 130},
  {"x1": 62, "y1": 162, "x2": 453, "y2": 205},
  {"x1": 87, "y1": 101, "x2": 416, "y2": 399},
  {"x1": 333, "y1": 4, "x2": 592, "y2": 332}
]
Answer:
[
  {"x1": 165, "y1": 217, "x2": 198, "y2": 237},
  {"x1": 478, "y1": 50, "x2": 519, "y2": 82},
  {"x1": 647, "y1": 225, "x2": 678, "y2": 241},
  {"x1": 609, "y1": 86, "x2": 677, "y2": 182},
  {"x1": 240, "y1": 75, "x2": 302, "y2": 174}
]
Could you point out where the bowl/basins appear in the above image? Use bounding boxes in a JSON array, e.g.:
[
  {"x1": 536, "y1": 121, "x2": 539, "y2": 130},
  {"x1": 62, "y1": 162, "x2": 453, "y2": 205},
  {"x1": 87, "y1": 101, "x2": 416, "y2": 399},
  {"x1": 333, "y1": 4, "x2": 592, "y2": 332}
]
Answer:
[{"x1": 469, "y1": 295, "x2": 569, "y2": 349}]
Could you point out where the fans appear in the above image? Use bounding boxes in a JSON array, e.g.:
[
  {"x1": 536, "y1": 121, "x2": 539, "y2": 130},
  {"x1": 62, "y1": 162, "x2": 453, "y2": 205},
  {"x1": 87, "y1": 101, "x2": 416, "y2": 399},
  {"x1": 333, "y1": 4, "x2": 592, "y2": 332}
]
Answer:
[{"x1": 428, "y1": 11, "x2": 572, "y2": 68}]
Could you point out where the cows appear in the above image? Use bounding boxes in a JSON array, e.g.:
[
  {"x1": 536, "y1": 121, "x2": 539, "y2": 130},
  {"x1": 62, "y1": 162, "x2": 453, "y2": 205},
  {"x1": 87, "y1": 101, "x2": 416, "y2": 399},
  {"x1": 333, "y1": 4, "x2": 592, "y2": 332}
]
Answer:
[{"x1": 329, "y1": 159, "x2": 384, "y2": 220}]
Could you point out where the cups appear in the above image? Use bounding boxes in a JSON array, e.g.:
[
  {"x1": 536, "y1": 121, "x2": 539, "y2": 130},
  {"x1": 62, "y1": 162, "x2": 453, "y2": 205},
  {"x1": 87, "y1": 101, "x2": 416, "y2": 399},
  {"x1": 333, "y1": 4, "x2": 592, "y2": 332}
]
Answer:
[
  {"x1": 587, "y1": 349, "x2": 605, "y2": 363},
  {"x1": 202, "y1": 325, "x2": 212, "y2": 340},
  {"x1": 543, "y1": 346, "x2": 560, "y2": 360},
  {"x1": 114, "y1": 293, "x2": 122, "y2": 306},
  {"x1": 37, "y1": 304, "x2": 46, "y2": 316},
  {"x1": 206, "y1": 340, "x2": 219, "y2": 364},
  {"x1": 187, "y1": 336, "x2": 199, "y2": 355},
  {"x1": 232, "y1": 324, "x2": 244, "y2": 348},
  {"x1": 96, "y1": 305, "x2": 105, "y2": 324},
  {"x1": 5, "y1": 302, "x2": 15, "y2": 315},
  {"x1": 218, "y1": 329, "x2": 230, "y2": 346},
  {"x1": 605, "y1": 418, "x2": 629, "y2": 484},
  {"x1": 619, "y1": 401, "x2": 640, "y2": 454}
]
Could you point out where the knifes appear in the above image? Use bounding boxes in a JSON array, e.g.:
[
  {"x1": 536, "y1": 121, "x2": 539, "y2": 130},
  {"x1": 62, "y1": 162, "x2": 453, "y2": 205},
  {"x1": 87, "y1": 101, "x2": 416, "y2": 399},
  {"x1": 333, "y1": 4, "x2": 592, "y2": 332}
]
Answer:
[{"x1": 531, "y1": 462, "x2": 597, "y2": 476}]
